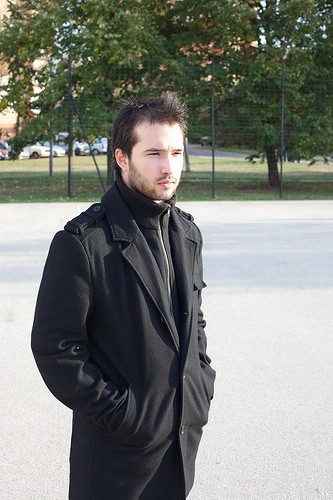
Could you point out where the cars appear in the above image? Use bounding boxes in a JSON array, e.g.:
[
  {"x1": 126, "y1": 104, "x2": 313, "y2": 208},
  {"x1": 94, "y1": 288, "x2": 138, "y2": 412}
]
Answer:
[{"x1": 0, "y1": 133, "x2": 107, "y2": 162}]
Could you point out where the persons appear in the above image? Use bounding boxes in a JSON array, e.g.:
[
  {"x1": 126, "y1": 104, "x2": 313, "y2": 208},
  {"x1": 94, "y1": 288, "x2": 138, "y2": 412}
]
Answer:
[{"x1": 31, "y1": 91, "x2": 216, "y2": 500}]
